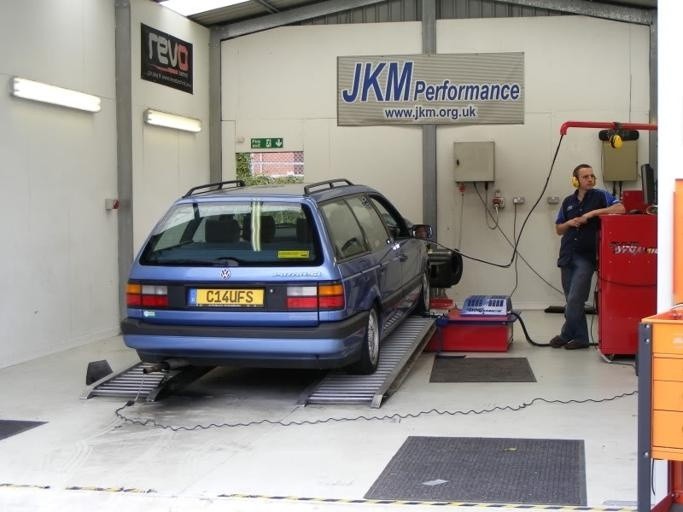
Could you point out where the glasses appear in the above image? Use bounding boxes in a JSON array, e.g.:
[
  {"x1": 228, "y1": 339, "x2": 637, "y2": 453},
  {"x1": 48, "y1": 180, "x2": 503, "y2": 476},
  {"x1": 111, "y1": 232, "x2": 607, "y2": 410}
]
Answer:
[
  {"x1": 143, "y1": 107, "x2": 203, "y2": 134},
  {"x1": 7, "y1": 74, "x2": 101, "y2": 114}
]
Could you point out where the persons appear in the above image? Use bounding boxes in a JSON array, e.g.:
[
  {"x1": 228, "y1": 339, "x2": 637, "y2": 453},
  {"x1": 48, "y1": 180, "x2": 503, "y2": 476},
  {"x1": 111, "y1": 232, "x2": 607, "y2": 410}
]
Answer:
[{"x1": 548, "y1": 162, "x2": 626, "y2": 350}]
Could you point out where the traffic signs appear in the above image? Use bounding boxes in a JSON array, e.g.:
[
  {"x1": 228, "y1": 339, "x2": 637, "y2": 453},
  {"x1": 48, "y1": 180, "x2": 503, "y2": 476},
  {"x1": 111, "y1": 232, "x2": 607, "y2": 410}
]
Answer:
[{"x1": 493, "y1": 190, "x2": 562, "y2": 208}]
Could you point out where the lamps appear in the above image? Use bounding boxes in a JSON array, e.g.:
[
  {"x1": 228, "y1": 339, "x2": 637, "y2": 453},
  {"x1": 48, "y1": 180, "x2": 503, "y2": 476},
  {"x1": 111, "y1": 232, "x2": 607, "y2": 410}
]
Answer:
[{"x1": 191, "y1": 213, "x2": 309, "y2": 250}]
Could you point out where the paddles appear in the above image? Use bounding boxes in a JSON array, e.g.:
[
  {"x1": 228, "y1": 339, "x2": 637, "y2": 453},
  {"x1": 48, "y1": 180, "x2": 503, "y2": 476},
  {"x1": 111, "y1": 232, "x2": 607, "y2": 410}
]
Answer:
[
  {"x1": 366, "y1": 435, "x2": 588, "y2": 509},
  {"x1": 428, "y1": 355, "x2": 538, "y2": 384}
]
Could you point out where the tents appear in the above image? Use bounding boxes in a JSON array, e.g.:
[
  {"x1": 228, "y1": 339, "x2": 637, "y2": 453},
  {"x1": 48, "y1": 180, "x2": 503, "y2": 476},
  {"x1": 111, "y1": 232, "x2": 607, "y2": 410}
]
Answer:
[
  {"x1": 609, "y1": 121, "x2": 624, "y2": 149},
  {"x1": 570, "y1": 166, "x2": 598, "y2": 188}
]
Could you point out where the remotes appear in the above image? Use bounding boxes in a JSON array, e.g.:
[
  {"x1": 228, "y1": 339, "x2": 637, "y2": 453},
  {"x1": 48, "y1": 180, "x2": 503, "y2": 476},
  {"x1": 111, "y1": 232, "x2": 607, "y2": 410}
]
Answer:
[{"x1": 629, "y1": 210, "x2": 640, "y2": 214}]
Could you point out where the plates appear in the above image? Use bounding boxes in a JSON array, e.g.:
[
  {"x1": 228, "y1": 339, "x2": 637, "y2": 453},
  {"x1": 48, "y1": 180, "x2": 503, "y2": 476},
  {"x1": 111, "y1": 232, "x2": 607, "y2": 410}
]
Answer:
[{"x1": 637, "y1": 308, "x2": 683, "y2": 512}]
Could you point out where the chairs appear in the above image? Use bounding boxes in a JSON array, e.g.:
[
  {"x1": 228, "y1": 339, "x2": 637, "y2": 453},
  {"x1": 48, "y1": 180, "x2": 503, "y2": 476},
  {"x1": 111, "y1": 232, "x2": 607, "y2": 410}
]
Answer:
[{"x1": 550, "y1": 336, "x2": 588, "y2": 349}]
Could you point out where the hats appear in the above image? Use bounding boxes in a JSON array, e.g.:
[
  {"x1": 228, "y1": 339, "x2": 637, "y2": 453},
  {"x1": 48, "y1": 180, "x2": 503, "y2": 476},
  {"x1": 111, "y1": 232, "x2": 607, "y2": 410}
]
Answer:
[{"x1": 118, "y1": 178, "x2": 437, "y2": 378}]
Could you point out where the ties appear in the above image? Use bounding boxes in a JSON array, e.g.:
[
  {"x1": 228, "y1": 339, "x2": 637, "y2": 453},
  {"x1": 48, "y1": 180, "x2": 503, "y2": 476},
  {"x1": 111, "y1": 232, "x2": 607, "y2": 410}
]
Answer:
[{"x1": 641, "y1": 163, "x2": 654, "y2": 205}]
